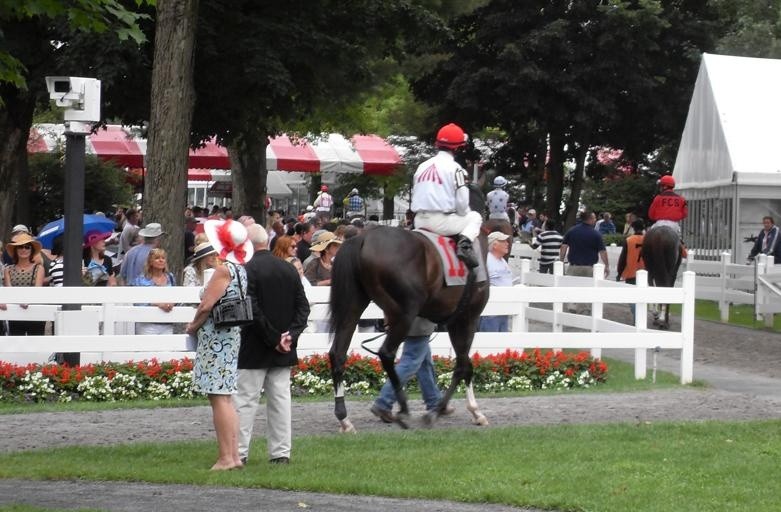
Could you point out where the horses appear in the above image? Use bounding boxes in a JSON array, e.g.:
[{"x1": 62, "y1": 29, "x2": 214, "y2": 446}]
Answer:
[
  {"x1": 480, "y1": 217, "x2": 516, "y2": 263},
  {"x1": 328, "y1": 183, "x2": 497, "y2": 430},
  {"x1": 640, "y1": 225, "x2": 683, "y2": 287}
]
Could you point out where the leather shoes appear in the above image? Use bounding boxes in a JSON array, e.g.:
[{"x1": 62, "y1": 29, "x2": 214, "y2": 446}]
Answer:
[
  {"x1": 439, "y1": 408, "x2": 456, "y2": 417},
  {"x1": 371, "y1": 404, "x2": 392, "y2": 423}
]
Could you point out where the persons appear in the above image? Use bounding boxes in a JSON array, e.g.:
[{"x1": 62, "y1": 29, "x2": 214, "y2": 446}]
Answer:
[
  {"x1": 183, "y1": 205, "x2": 232, "y2": 258},
  {"x1": 478, "y1": 203, "x2": 646, "y2": 333},
  {"x1": 313, "y1": 185, "x2": 334, "y2": 219},
  {"x1": 485, "y1": 176, "x2": 511, "y2": 224},
  {"x1": 746, "y1": 216, "x2": 781, "y2": 264},
  {"x1": 267, "y1": 203, "x2": 380, "y2": 286},
  {"x1": 1, "y1": 206, "x2": 218, "y2": 335},
  {"x1": 234, "y1": 222, "x2": 311, "y2": 465},
  {"x1": 410, "y1": 123, "x2": 483, "y2": 267},
  {"x1": 648, "y1": 175, "x2": 688, "y2": 258},
  {"x1": 185, "y1": 217, "x2": 255, "y2": 471},
  {"x1": 371, "y1": 311, "x2": 456, "y2": 423},
  {"x1": 343, "y1": 188, "x2": 366, "y2": 219}
]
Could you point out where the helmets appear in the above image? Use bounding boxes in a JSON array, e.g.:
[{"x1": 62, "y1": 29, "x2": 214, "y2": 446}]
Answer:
[
  {"x1": 436, "y1": 123, "x2": 468, "y2": 149},
  {"x1": 661, "y1": 175, "x2": 674, "y2": 187},
  {"x1": 494, "y1": 176, "x2": 507, "y2": 187}
]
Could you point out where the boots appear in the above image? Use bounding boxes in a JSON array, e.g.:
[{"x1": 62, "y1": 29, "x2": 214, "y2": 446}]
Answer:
[{"x1": 456, "y1": 235, "x2": 478, "y2": 267}]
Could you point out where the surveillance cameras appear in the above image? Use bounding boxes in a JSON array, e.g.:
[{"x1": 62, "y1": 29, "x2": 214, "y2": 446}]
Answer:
[{"x1": 45, "y1": 76, "x2": 97, "y2": 100}]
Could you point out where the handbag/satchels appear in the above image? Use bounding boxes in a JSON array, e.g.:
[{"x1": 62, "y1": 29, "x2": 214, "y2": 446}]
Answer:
[{"x1": 211, "y1": 295, "x2": 254, "y2": 327}]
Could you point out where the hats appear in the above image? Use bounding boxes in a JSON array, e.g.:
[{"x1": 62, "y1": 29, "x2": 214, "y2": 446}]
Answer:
[
  {"x1": 204, "y1": 219, "x2": 254, "y2": 264},
  {"x1": 191, "y1": 242, "x2": 215, "y2": 262},
  {"x1": 488, "y1": 231, "x2": 509, "y2": 244},
  {"x1": 84, "y1": 232, "x2": 111, "y2": 247},
  {"x1": 6, "y1": 233, "x2": 42, "y2": 261},
  {"x1": 137, "y1": 223, "x2": 165, "y2": 238},
  {"x1": 11, "y1": 225, "x2": 28, "y2": 233},
  {"x1": 309, "y1": 230, "x2": 342, "y2": 252}
]
